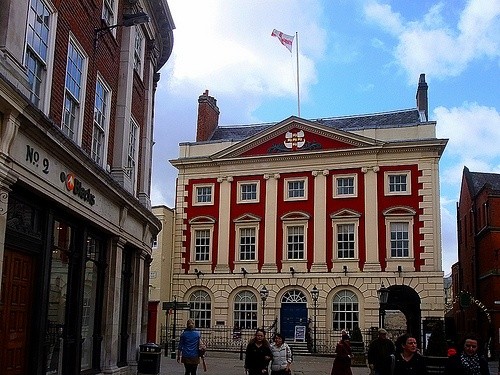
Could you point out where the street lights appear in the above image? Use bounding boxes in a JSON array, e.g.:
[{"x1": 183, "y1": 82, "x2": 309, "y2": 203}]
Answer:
[
  {"x1": 259, "y1": 284, "x2": 269, "y2": 331},
  {"x1": 309, "y1": 285, "x2": 320, "y2": 354},
  {"x1": 376, "y1": 281, "x2": 391, "y2": 329}
]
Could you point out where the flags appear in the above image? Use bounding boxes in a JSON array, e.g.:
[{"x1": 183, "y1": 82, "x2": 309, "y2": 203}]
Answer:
[{"x1": 271, "y1": 29, "x2": 295, "y2": 53}]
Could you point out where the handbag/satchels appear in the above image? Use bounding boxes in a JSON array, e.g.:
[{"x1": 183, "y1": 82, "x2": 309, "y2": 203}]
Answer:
[{"x1": 198, "y1": 338, "x2": 206, "y2": 357}]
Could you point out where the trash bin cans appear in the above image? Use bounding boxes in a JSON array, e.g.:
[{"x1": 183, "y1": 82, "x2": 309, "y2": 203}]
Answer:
[{"x1": 137, "y1": 342, "x2": 162, "y2": 375}]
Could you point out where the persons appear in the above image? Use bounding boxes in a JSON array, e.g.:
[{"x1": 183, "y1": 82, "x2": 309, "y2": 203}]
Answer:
[
  {"x1": 331, "y1": 340, "x2": 352, "y2": 375},
  {"x1": 177, "y1": 319, "x2": 206, "y2": 375},
  {"x1": 265, "y1": 333, "x2": 293, "y2": 375},
  {"x1": 443, "y1": 332, "x2": 490, "y2": 375},
  {"x1": 244, "y1": 329, "x2": 273, "y2": 375},
  {"x1": 368, "y1": 329, "x2": 395, "y2": 375},
  {"x1": 395, "y1": 334, "x2": 426, "y2": 375},
  {"x1": 341, "y1": 329, "x2": 350, "y2": 343}
]
